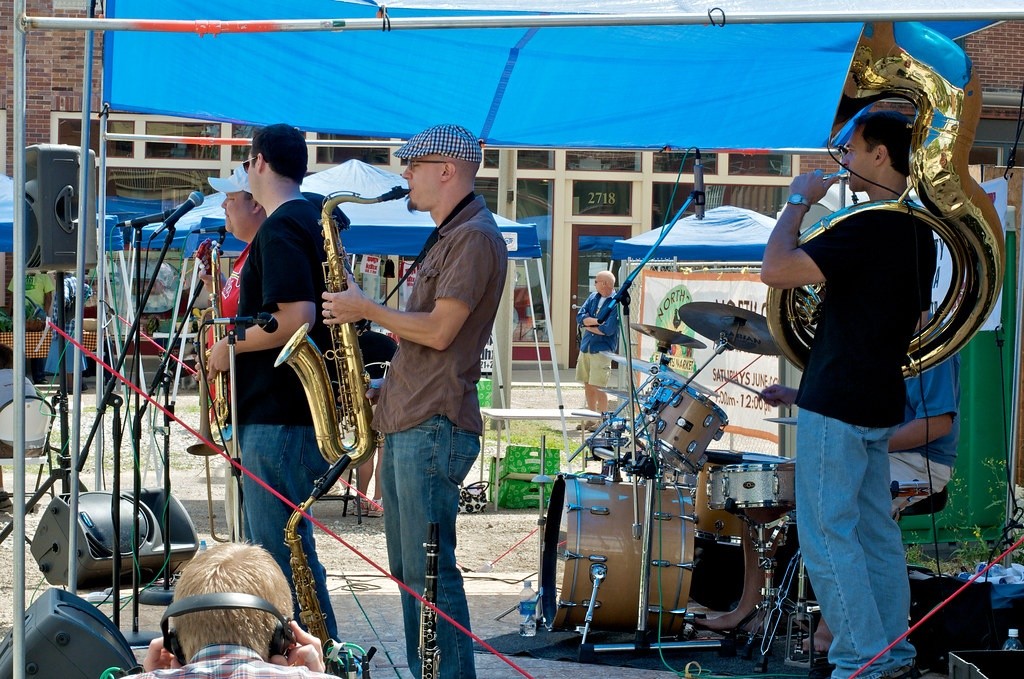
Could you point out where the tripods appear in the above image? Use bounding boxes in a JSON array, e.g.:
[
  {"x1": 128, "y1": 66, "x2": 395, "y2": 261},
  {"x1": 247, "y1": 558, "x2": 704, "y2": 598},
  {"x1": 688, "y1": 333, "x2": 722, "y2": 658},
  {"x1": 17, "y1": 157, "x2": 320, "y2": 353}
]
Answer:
[{"x1": 724, "y1": 510, "x2": 811, "y2": 659}]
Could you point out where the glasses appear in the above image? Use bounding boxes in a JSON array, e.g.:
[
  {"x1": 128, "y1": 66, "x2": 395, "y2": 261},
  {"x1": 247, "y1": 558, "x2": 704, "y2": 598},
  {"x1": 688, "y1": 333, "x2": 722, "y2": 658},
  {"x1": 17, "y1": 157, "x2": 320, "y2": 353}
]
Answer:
[
  {"x1": 407, "y1": 160, "x2": 448, "y2": 170},
  {"x1": 595, "y1": 280, "x2": 603, "y2": 284},
  {"x1": 242, "y1": 156, "x2": 259, "y2": 174}
]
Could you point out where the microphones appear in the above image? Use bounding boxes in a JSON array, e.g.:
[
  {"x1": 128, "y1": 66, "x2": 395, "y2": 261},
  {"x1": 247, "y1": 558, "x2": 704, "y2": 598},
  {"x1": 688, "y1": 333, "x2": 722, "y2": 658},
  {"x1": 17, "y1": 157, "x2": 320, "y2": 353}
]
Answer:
[
  {"x1": 192, "y1": 226, "x2": 228, "y2": 233},
  {"x1": 117, "y1": 209, "x2": 178, "y2": 228},
  {"x1": 151, "y1": 191, "x2": 205, "y2": 240},
  {"x1": 694, "y1": 148, "x2": 705, "y2": 221}
]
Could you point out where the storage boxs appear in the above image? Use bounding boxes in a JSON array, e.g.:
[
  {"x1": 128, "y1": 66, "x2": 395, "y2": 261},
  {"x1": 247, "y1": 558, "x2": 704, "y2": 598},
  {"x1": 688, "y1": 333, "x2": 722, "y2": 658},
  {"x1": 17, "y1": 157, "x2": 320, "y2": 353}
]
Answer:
[
  {"x1": 488, "y1": 445, "x2": 560, "y2": 509},
  {"x1": 477, "y1": 377, "x2": 493, "y2": 408}
]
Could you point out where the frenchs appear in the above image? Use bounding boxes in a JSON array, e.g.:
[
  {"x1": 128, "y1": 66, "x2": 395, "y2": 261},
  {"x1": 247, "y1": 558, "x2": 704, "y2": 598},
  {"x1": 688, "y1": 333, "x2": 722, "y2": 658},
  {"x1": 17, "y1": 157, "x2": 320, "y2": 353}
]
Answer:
[{"x1": 766, "y1": 20, "x2": 1007, "y2": 384}]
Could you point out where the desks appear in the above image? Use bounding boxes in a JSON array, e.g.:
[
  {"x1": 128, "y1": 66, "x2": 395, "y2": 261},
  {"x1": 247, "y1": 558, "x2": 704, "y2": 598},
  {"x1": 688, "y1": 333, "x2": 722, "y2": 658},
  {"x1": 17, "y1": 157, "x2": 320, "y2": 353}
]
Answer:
[
  {"x1": 0, "y1": 329, "x2": 96, "y2": 394},
  {"x1": 480, "y1": 406, "x2": 602, "y2": 513},
  {"x1": 152, "y1": 332, "x2": 199, "y2": 389}
]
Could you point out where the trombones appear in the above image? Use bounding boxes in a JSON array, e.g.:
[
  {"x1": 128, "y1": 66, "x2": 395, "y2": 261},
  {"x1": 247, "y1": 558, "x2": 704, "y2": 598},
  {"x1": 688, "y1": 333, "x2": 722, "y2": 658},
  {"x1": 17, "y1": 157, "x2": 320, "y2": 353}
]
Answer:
[
  {"x1": 194, "y1": 231, "x2": 230, "y2": 429},
  {"x1": 186, "y1": 290, "x2": 232, "y2": 544}
]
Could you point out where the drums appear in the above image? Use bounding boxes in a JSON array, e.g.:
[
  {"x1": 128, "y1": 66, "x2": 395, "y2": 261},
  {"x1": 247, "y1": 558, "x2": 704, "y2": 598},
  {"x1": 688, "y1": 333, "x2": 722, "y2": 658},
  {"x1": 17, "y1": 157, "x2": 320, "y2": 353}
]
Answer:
[
  {"x1": 538, "y1": 472, "x2": 693, "y2": 637},
  {"x1": 705, "y1": 462, "x2": 796, "y2": 511},
  {"x1": 634, "y1": 376, "x2": 729, "y2": 474},
  {"x1": 693, "y1": 449, "x2": 791, "y2": 546}
]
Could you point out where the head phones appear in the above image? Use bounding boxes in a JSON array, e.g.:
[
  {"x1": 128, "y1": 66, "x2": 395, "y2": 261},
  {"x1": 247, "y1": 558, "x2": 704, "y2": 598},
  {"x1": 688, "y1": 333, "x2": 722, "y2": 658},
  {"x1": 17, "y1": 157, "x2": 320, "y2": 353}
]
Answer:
[{"x1": 159, "y1": 592, "x2": 297, "y2": 667}]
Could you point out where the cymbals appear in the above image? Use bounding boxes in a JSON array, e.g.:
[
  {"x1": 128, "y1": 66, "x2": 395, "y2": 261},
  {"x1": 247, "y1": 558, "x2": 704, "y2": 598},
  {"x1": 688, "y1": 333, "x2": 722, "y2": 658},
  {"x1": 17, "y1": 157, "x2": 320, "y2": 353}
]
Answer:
[
  {"x1": 599, "y1": 350, "x2": 722, "y2": 398},
  {"x1": 678, "y1": 301, "x2": 783, "y2": 356},
  {"x1": 763, "y1": 418, "x2": 798, "y2": 425},
  {"x1": 596, "y1": 389, "x2": 650, "y2": 405},
  {"x1": 630, "y1": 323, "x2": 708, "y2": 350}
]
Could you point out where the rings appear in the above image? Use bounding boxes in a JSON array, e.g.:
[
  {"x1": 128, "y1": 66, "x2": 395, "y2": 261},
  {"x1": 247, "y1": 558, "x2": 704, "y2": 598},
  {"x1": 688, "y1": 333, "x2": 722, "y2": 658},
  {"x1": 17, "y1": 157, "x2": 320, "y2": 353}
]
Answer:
[{"x1": 325, "y1": 309, "x2": 332, "y2": 318}]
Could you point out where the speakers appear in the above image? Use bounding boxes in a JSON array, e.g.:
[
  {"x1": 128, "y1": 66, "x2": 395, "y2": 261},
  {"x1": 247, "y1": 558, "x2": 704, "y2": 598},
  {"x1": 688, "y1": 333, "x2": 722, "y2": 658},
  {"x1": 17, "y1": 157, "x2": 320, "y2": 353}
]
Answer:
[
  {"x1": 0, "y1": 588, "x2": 138, "y2": 679},
  {"x1": 25, "y1": 143, "x2": 97, "y2": 277},
  {"x1": 29, "y1": 488, "x2": 199, "y2": 592}
]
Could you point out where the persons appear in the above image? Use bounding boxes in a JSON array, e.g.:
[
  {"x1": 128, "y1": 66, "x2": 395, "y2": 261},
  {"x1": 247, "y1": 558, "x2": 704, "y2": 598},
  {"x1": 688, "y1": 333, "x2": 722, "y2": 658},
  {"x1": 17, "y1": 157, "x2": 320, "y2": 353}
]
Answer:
[
  {"x1": 356, "y1": 332, "x2": 397, "y2": 516},
  {"x1": 196, "y1": 122, "x2": 349, "y2": 679},
  {"x1": 575, "y1": 270, "x2": 621, "y2": 432},
  {"x1": 44, "y1": 266, "x2": 92, "y2": 396},
  {"x1": 695, "y1": 308, "x2": 961, "y2": 656},
  {"x1": 0, "y1": 343, "x2": 45, "y2": 512},
  {"x1": 760, "y1": 109, "x2": 938, "y2": 679},
  {"x1": 8, "y1": 270, "x2": 55, "y2": 384},
  {"x1": 322, "y1": 124, "x2": 508, "y2": 679},
  {"x1": 116, "y1": 539, "x2": 344, "y2": 679}
]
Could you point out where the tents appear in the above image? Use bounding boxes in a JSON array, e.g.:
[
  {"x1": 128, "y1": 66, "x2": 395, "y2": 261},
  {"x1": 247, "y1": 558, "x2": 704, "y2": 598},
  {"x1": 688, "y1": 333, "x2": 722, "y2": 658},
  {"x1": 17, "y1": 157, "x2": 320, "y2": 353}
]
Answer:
[
  {"x1": 513, "y1": 215, "x2": 624, "y2": 303},
  {"x1": 0, "y1": 159, "x2": 573, "y2": 476},
  {"x1": 611, "y1": 206, "x2": 802, "y2": 459}
]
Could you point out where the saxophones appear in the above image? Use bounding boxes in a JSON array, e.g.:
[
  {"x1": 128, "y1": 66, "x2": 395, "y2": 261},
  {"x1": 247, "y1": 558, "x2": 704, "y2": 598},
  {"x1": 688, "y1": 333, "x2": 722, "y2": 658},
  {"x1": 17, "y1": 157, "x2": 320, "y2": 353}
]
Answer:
[
  {"x1": 274, "y1": 185, "x2": 413, "y2": 470},
  {"x1": 284, "y1": 453, "x2": 353, "y2": 675}
]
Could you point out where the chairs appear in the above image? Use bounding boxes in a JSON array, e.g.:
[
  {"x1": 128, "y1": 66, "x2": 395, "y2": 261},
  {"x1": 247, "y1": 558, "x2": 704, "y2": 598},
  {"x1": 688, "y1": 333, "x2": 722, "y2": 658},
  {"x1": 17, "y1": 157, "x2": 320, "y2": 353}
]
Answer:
[{"x1": 0, "y1": 396, "x2": 56, "y2": 514}]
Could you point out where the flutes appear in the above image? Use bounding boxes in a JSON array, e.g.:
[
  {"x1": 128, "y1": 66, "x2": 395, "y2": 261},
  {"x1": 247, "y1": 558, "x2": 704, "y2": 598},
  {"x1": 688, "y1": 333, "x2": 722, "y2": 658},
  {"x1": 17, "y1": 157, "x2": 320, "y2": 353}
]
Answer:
[{"x1": 418, "y1": 519, "x2": 442, "y2": 678}]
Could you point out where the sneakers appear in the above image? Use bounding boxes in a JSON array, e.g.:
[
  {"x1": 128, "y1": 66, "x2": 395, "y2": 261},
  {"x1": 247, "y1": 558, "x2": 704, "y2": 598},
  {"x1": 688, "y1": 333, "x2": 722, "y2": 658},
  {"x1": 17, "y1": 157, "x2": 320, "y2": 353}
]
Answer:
[{"x1": 576, "y1": 420, "x2": 602, "y2": 432}]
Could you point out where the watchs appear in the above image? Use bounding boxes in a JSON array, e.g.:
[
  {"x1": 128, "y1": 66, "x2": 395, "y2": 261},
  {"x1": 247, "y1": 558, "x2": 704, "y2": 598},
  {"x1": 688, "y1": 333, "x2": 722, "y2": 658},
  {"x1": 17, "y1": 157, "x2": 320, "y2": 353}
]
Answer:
[{"x1": 787, "y1": 192, "x2": 811, "y2": 212}]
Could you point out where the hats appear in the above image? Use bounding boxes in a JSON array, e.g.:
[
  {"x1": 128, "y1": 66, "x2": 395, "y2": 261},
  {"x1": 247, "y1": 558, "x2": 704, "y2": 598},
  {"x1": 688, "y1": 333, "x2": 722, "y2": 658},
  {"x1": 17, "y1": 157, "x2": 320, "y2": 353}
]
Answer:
[
  {"x1": 392, "y1": 124, "x2": 485, "y2": 164},
  {"x1": 207, "y1": 166, "x2": 253, "y2": 196}
]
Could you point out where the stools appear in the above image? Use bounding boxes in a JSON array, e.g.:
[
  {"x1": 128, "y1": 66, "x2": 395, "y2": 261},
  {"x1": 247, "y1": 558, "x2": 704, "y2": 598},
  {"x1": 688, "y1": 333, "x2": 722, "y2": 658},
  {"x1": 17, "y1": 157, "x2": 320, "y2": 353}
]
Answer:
[{"x1": 899, "y1": 485, "x2": 949, "y2": 517}]
